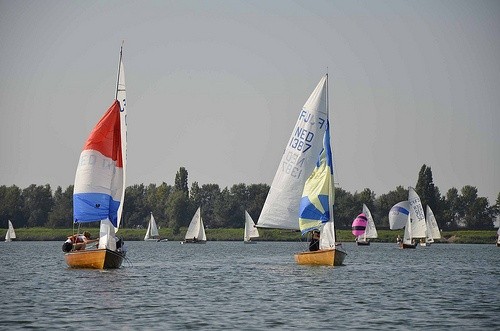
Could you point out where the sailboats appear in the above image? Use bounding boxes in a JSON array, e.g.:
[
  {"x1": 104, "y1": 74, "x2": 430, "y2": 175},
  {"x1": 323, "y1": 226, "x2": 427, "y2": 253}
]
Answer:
[
  {"x1": 144, "y1": 211, "x2": 168, "y2": 242},
  {"x1": 389, "y1": 185, "x2": 428, "y2": 249},
  {"x1": 252, "y1": 66, "x2": 348, "y2": 266},
  {"x1": 351, "y1": 203, "x2": 378, "y2": 246},
  {"x1": 4, "y1": 220, "x2": 16, "y2": 242},
  {"x1": 185, "y1": 206, "x2": 208, "y2": 243},
  {"x1": 62, "y1": 39, "x2": 129, "y2": 270},
  {"x1": 420, "y1": 204, "x2": 441, "y2": 247},
  {"x1": 244, "y1": 210, "x2": 259, "y2": 244}
]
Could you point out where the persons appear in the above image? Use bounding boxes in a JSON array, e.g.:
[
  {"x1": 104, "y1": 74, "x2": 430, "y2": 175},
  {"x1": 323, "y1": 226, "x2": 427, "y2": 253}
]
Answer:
[
  {"x1": 397, "y1": 234, "x2": 402, "y2": 245},
  {"x1": 309, "y1": 229, "x2": 320, "y2": 251},
  {"x1": 62, "y1": 231, "x2": 99, "y2": 253}
]
